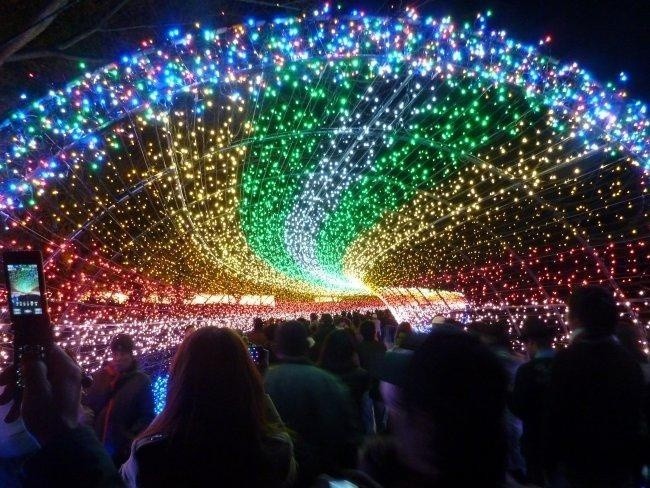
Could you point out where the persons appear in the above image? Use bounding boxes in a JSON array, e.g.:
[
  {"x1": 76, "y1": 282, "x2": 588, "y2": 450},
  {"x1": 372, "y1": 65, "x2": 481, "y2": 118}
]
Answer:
[{"x1": 1, "y1": 284, "x2": 650, "y2": 488}]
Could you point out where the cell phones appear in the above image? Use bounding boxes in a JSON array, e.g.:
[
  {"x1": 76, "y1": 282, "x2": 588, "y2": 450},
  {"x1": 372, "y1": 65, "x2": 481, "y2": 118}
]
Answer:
[{"x1": 3, "y1": 251, "x2": 52, "y2": 394}]
